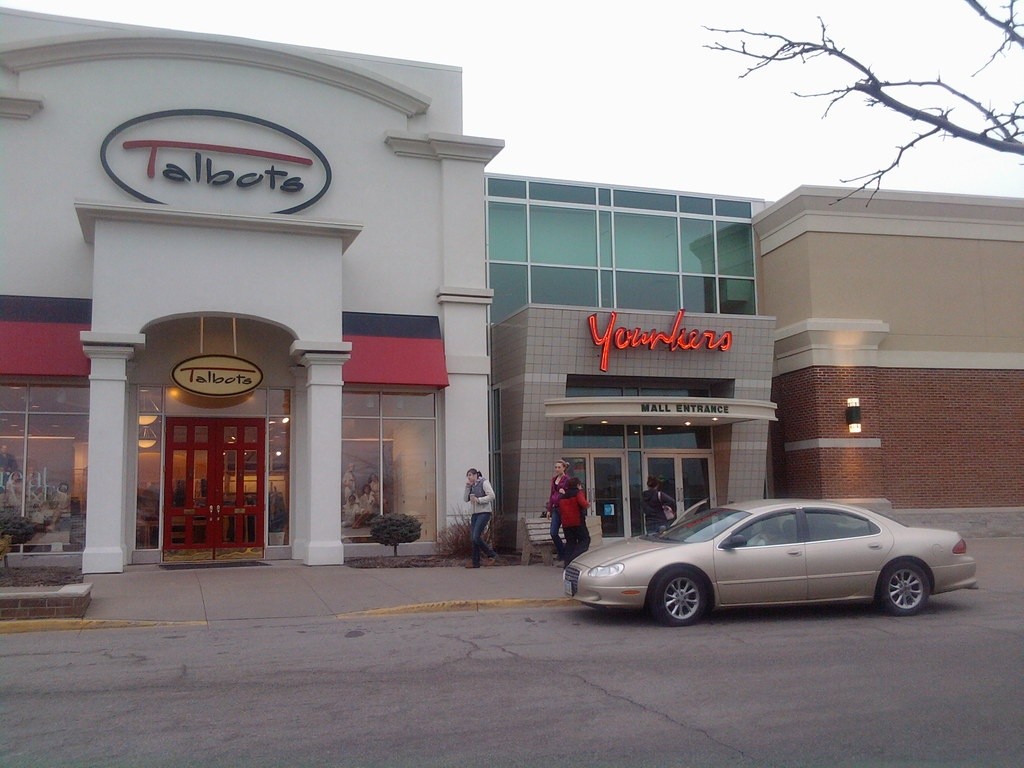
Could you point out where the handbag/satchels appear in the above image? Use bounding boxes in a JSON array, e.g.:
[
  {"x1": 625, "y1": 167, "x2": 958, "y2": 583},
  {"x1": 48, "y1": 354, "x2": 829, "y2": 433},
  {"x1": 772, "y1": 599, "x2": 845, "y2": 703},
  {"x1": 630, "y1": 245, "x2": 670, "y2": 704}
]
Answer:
[{"x1": 662, "y1": 505, "x2": 675, "y2": 520}]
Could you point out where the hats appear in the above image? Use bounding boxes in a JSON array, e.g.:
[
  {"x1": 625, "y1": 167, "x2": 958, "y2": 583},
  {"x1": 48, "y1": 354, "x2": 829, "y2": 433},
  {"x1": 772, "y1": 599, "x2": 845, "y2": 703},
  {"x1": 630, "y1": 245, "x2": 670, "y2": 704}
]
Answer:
[
  {"x1": 647, "y1": 476, "x2": 657, "y2": 487},
  {"x1": 564, "y1": 477, "x2": 584, "y2": 486}
]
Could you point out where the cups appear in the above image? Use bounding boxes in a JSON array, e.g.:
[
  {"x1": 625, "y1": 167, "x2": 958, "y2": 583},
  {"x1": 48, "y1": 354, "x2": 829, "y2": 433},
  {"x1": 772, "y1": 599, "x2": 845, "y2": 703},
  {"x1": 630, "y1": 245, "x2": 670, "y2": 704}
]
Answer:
[{"x1": 470, "y1": 494, "x2": 475, "y2": 504}]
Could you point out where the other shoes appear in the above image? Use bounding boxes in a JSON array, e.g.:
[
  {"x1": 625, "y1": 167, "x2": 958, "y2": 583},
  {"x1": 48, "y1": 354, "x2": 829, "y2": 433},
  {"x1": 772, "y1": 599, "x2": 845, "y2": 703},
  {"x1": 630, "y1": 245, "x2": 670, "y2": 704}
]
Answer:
[
  {"x1": 556, "y1": 551, "x2": 562, "y2": 560},
  {"x1": 493, "y1": 554, "x2": 500, "y2": 563},
  {"x1": 465, "y1": 563, "x2": 480, "y2": 568}
]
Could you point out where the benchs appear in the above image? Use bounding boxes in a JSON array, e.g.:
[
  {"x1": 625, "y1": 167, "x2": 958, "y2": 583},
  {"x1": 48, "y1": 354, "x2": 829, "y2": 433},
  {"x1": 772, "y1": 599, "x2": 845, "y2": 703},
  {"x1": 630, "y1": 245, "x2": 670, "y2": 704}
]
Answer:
[
  {"x1": 8, "y1": 532, "x2": 71, "y2": 553},
  {"x1": 520, "y1": 517, "x2": 567, "y2": 567}
]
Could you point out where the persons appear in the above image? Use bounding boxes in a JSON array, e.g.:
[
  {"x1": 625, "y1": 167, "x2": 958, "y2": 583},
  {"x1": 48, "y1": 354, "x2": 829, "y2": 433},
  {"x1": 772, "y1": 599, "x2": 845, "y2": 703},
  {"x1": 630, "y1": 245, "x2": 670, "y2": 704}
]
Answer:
[
  {"x1": 0, "y1": 443, "x2": 18, "y2": 470},
  {"x1": 463, "y1": 467, "x2": 499, "y2": 570},
  {"x1": 343, "y1": 464, "x2": 388, "y2": 532},
  {"x1": 544, "y1": 460, "x2": 591, "y2": 570},
  {"x1": 637, "y1": 476, "x2": 676, "y2": 534}
]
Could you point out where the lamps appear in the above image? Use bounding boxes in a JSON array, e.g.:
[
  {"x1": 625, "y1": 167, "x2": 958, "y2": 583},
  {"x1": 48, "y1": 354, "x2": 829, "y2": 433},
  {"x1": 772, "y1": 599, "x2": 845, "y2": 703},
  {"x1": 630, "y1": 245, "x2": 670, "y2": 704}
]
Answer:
[
  {"x1": 138, "y1": 390, "x2": 160, "y2": 425},
  {"x1": 846, "y1": 398, "x2": 861, "y2": 434},
  {"x1": 138, "y1": 426, "x2": 158, "y2": 448}
]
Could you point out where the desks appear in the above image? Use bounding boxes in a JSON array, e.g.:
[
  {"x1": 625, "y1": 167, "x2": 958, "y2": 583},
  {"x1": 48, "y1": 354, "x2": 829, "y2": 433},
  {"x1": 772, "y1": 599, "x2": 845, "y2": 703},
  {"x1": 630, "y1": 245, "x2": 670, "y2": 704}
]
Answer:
[{"x1": 137, "y1": 519, "x2": 207, "y2": 548}]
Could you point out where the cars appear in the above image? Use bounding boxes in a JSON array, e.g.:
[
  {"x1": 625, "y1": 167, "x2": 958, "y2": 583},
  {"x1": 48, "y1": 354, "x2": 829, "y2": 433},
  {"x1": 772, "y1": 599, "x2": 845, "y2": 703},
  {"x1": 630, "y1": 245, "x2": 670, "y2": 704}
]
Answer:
[{"x1": 561, "y1": 498, "x2": 979, "y2": 628}]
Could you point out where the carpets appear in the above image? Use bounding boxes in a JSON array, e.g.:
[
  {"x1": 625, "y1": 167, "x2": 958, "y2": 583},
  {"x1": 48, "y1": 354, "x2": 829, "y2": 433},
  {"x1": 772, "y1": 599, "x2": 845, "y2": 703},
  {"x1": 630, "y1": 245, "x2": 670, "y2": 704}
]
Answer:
[{"x1": 159, "y1": 560, "x2": 272, "y2": 570}]
